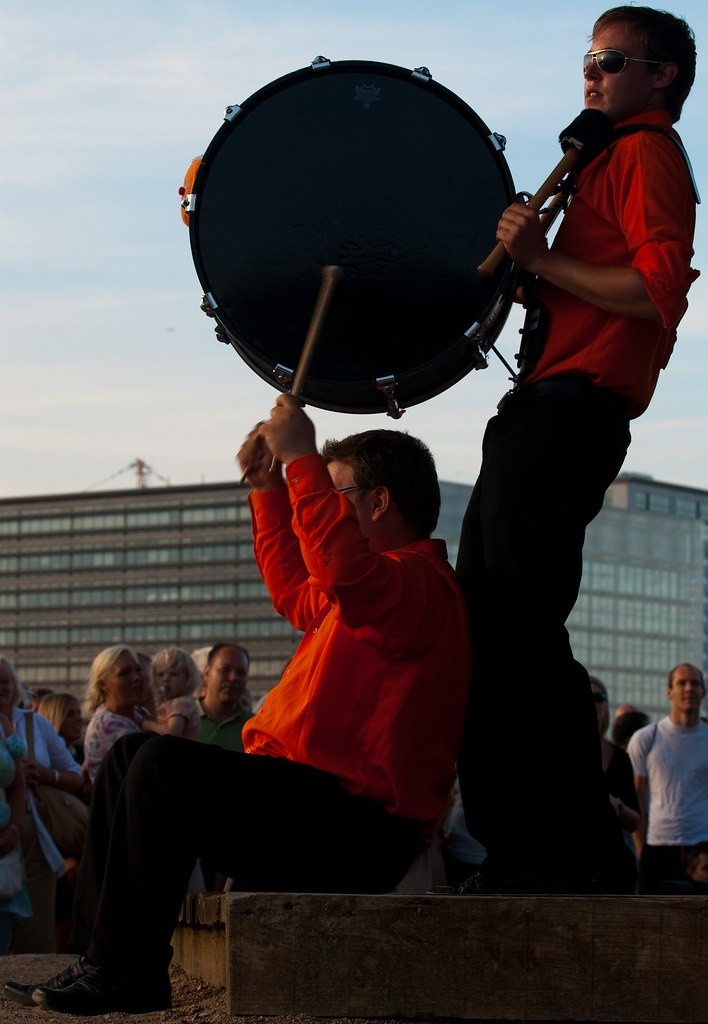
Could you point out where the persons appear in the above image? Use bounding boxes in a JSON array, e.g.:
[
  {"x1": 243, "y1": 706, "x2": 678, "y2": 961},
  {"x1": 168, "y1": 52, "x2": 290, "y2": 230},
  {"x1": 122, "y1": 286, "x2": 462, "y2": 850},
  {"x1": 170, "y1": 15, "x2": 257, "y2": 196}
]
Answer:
[
  {"x1": 457, "y1": 6, "x2": 700, "y2": 896},
  {"x1": 0, "y1": 645, "x2": 257, "y2": 955},
  {"x1": 628, "y1": 663, "x2": 705, "y2": 894},
  {"x1": 589, "y1": 677, "x2": 642, "y2": 895},
  {"x1": 686, "y1": 841, "x2": 708, "y2": 883},
  {"x1": 4, "y1": 393, "x2": 470, "y2": 1016}
]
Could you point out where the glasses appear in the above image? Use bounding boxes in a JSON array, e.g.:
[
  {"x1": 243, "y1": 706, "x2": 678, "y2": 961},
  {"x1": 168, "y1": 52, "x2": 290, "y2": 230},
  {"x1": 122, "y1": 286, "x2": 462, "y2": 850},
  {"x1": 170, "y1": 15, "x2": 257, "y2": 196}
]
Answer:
[
  {"x1": 594, "y1": 692, "x2": 606, "y2": 704},
  {"x1": 338, "y1": 482, "x2": 377, "y2": 493},
  {"x1": 583, "y1": 50, "x2": 668, "y2": 73}
]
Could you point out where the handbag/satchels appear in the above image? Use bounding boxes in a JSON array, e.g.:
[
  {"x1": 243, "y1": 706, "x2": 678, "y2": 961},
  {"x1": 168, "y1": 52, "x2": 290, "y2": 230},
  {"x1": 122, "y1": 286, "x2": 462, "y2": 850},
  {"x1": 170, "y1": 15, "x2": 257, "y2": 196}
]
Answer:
[{"x1": 24, "y1": 712, "x2": 89, "y2": 858}]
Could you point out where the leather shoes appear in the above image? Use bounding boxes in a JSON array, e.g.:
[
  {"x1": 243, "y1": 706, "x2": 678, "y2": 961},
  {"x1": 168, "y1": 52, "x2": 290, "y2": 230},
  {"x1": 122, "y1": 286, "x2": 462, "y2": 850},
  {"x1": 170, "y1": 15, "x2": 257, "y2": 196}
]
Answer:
[{"x1": 5, "y1": 956, "x2": 171, "y2": 1015}]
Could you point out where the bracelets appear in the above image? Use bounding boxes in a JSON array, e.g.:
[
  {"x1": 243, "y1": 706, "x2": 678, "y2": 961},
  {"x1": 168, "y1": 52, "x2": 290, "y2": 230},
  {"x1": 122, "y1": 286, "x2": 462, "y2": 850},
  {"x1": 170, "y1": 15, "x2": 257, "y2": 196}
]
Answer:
[{"x1": 9, "y1": 824, "x2": 21, "y2": 841}]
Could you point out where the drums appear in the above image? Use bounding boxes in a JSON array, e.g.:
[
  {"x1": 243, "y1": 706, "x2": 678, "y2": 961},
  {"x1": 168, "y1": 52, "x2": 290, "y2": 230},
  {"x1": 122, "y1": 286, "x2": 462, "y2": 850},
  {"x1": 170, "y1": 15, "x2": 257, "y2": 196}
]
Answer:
[{"x1": 184, "y1": 53, "x2": 524, "y2": 422}]
